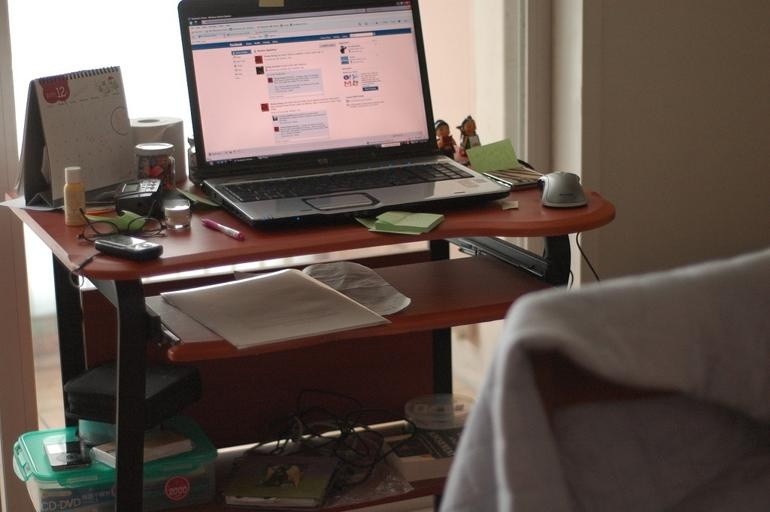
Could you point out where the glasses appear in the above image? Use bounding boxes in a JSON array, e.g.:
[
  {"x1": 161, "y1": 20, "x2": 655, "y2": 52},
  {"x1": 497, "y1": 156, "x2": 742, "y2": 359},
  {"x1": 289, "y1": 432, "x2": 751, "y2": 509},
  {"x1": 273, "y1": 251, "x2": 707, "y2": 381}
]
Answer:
[{"x1": 76, "y1": 200, "x2": 167, "y2": 242}]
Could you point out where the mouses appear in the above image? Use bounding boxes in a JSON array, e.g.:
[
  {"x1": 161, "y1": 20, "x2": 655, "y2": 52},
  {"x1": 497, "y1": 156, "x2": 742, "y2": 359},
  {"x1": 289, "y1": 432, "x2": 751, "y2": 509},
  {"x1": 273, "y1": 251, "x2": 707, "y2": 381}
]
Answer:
[{"x1": 538, "y1": 171, "x2": 587, "y2": 210}]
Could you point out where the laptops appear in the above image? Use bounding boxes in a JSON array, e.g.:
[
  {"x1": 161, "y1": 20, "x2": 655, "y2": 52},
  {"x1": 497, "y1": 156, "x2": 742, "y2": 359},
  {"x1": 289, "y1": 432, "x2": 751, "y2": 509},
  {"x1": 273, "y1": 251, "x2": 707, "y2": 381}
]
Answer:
[{"x1": 178, "y1": 0, "x2": 512, "y2": 230}]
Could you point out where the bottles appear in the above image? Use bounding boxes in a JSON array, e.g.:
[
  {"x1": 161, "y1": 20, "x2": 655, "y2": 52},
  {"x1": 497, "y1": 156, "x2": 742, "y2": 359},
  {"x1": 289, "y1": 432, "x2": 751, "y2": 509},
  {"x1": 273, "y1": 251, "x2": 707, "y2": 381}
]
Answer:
[
  {"x1": 62, "y1": 165, "x2": 86, "y2": 230},
  {"x1": 133, "y1": 140, "x2": 176, "y2": 198}
]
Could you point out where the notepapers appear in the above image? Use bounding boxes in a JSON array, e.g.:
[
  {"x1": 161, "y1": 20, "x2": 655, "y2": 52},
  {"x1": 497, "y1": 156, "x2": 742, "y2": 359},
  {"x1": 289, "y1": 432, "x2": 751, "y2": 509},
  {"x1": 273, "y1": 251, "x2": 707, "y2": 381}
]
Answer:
[{"x1": 368, "y1": 211, "x2": 444, "y2": 235}]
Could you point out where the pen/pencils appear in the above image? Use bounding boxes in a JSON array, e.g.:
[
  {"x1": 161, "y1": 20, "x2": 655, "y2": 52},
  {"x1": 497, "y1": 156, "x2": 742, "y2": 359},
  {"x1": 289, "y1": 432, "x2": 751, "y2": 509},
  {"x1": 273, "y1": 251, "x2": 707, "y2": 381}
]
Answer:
[
  {"x1": 200, "y1": 216, "x2": 244, "y2": 241},
  {"x1": 481, "y1": 168, "x2": 544, "y2": 186}
]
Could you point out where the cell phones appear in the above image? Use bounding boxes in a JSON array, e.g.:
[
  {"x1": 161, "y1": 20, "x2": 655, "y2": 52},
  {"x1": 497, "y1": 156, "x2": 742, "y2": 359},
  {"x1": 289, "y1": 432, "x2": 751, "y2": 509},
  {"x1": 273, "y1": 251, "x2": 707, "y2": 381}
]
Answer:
[
  {"x1": 43, "y1": 433, "x2": 92, "y2": 471},
  {"x1": 94, "y1": 234, "x2": 162, "y2": 259}
]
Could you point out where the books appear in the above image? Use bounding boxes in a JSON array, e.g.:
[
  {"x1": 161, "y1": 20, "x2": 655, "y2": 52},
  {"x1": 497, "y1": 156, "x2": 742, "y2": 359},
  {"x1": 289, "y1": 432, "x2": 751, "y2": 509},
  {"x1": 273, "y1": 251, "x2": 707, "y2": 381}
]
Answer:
[
  {"x1": 218, "y1": 448, "x2": 344, "y2": 506},
  {"x1": 377, "y1": 421, "x2": 464, "y2": 484}
]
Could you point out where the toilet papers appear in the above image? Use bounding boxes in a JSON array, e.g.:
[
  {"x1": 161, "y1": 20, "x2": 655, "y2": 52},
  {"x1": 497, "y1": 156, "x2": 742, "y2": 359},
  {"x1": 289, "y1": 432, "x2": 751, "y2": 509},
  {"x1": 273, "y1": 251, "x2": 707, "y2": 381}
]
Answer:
[{"x1": 129, "y1": 116, "x2": 186, "y2": 182}]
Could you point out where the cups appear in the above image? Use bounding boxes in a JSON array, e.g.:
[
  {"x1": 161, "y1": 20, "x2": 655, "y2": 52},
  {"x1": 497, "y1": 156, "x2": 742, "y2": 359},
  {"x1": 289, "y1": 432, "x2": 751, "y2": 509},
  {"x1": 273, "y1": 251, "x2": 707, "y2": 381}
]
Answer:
[{"x1": 162, "y1": 197, "x2": 192, "y2": 233}]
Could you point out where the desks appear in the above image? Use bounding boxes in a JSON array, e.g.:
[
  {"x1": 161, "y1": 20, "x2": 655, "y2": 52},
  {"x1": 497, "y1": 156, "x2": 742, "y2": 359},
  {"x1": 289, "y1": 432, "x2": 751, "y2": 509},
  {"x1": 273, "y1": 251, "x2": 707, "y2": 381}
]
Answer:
[{"x1": 1, "y1": 165, "x2": 617, "y2": 510}]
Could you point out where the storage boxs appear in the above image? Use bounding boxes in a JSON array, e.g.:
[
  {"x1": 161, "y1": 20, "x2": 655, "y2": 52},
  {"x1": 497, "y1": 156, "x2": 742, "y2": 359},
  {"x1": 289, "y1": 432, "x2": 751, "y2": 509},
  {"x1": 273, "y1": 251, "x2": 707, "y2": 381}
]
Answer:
[{"x1": 11, "y1": 414, "x2": 218, "y2": 510}]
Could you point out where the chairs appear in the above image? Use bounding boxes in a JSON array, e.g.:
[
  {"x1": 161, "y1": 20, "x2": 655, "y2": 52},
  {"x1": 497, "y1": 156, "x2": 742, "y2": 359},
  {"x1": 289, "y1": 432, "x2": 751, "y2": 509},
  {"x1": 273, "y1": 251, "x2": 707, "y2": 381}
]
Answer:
[{"x1": 428, "y1": 243, "x2": 770, "y2": 511}]
